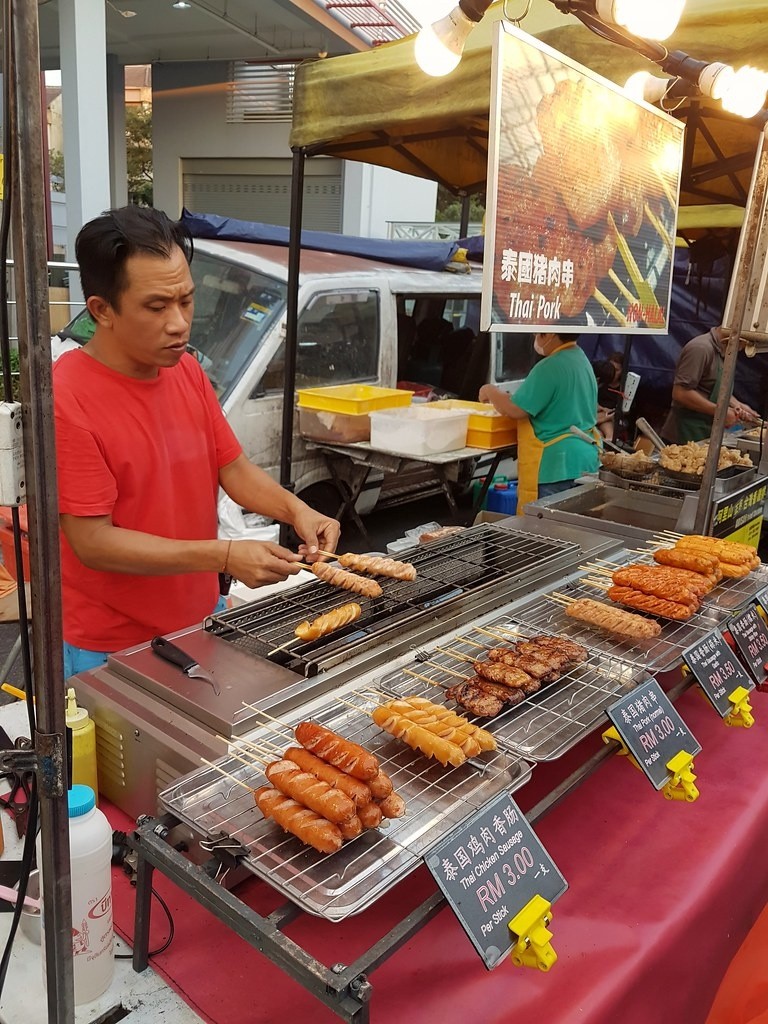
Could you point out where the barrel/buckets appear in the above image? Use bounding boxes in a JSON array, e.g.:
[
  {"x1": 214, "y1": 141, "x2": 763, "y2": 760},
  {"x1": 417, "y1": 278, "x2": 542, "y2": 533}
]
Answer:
[
  {"x1": 473, "y1": 474, "x2": 509, "y2": 510},
  {"x1": 487, "y1": 479, "x2": 520, "y2": 516}
]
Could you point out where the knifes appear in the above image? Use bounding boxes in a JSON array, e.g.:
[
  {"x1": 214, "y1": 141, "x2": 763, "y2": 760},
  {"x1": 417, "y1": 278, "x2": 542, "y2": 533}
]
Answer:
[{"x1": 151, "y1": 635, "x2": 222, "y2": 696}]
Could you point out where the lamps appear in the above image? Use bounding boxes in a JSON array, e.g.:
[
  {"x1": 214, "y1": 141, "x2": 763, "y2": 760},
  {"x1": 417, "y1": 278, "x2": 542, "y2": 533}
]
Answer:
[{"x1": 173, "y1": 0, "x2": 191, "y2": 9}]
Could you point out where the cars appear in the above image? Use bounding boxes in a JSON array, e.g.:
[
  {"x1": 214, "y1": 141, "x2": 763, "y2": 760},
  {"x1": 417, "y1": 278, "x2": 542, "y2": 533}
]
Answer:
[{"x1": 23, "y1": 236, "x2": 528, "y2": 586}]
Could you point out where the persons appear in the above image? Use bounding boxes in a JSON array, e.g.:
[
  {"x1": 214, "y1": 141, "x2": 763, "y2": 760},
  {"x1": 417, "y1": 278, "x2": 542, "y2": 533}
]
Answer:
[
  {"x1": 31, "y1": 206, "x2": 341, "y2": 684},
  {"x1": 479, "y1": 331, "x2": 626, "y2": 522},
  {"x1": 659, "y1": 325, "x2": 761, "y2": 446}
]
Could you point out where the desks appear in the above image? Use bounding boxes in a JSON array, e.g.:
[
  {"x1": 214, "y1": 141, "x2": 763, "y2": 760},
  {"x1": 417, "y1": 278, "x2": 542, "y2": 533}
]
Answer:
[
  {"x1": 0, "y1": 633, "x2": 767, "y2": 1024},
  {"x1": 303, "y1": 437, "x2": 518, "y2": 554}
]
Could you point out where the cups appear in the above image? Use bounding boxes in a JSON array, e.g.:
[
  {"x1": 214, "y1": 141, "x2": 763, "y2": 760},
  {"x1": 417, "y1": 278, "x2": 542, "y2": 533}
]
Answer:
[{"x1": 11, "y1": 868, "x2": 41, "y2": 945}]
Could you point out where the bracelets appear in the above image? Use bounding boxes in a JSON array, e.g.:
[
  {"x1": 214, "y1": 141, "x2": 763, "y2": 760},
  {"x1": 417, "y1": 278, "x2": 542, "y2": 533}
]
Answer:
[{"x1": 224, "y1": 540, "x2": 231, "y2": 574}]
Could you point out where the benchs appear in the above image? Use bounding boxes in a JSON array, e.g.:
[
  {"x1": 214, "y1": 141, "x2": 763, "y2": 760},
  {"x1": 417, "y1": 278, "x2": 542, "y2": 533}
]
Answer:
[{"x1": 372, "y1": 311, "x2": 492, "y2": 400}]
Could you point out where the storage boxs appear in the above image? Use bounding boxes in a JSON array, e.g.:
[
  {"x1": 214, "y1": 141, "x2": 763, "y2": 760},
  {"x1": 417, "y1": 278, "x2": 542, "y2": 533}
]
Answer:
[
  {"x1": 423, "y1": 398, "x2": 518, "y2": 449},
  {"x1": 296, "y1": 406, "x2": 372, "y2": 444},
  {"x1": 367, "y1": 404, "x2": 471, "y2": 456},
  {"x1": 294, "y1": 383, "x2": 416, "y2": 417}
]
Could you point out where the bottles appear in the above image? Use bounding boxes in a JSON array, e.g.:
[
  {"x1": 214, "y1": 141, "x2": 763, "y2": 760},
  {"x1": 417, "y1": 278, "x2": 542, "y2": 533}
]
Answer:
[
  {"x1": 64, "y1": 687, "x2": 98, "y2": 809},
  {"x1": 36, "y1": 783, "x2": 114, "y2": 1004}
]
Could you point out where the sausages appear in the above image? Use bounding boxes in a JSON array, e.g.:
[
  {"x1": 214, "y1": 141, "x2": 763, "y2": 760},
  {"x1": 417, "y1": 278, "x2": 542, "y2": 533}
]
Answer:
[
  {"x1": 294, "y1": 602, "x2": 361, "y2": 641},
  {"x1": 256, "y1": 721, "x2": 406, "y2": 855},
  {"x1": 339, "y1": 551, "x2": 417, "y2": 582},
  {"x1": 565, "y1": 534, "x2": 760, "y2": 640},
  {"x1": 311, "y1": 562, "x2": 383, "y2": 598},
  {"x1": 371, "y1": 695, "x2": 497, "y2": 768}
]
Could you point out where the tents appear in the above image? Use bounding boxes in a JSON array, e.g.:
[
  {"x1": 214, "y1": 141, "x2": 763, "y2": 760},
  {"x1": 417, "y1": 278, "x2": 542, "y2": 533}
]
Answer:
[{"x1": 280, "y1": 1, "x2": 768, "y2": 547}]
